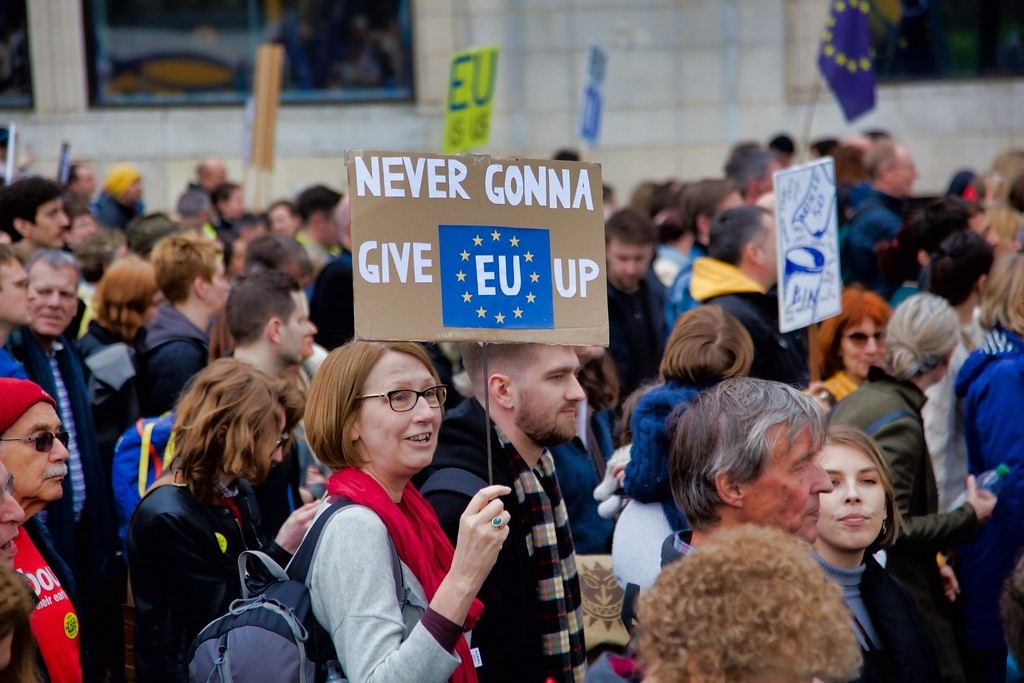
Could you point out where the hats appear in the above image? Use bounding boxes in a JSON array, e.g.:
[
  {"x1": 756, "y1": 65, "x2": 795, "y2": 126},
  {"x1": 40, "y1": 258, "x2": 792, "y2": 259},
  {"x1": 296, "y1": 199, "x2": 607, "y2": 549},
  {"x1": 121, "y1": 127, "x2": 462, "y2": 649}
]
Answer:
[
  {"x1": 104, "y1": 165, "x2": 140, "y2": 201},
  {"x1": 0, "y1": 378, "x2": 56, "y2": 439}
]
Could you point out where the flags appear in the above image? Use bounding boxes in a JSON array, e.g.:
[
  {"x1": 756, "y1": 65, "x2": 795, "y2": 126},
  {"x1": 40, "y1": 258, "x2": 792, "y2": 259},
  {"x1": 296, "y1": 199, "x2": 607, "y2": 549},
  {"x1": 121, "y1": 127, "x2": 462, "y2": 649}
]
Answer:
[{"x1": 818, "y1": 0, "x2": 875, "y2": 123}]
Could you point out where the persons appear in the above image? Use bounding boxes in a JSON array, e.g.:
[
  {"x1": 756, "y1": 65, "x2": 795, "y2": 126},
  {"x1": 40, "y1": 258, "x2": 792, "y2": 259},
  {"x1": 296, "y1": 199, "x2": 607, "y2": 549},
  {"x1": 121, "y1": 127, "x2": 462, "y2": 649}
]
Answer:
[
  {"x1": 636, "y1": 524, "x2": 864, "y2": 683},
  {"x1": 303, "y1": 341, "x2": 511, "y2": 683},
  {"x1": 0, "y1": 129, "x2": 1023, "y2": 683}
]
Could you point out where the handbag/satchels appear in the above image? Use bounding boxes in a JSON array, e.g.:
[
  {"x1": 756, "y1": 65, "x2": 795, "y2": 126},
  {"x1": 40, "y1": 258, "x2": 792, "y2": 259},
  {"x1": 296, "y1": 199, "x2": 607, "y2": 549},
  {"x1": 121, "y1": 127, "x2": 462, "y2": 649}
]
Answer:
[{"x1": 574, "y1": 554, "x2": 640, "y2": 649}]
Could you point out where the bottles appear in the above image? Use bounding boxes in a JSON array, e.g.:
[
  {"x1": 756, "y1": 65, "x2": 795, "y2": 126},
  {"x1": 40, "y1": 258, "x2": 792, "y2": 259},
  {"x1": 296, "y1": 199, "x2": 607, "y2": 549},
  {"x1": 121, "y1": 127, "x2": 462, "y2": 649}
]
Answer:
[
  {"x1": 946, "y1": 464, "x2": 1009, "y2": 514},
  {"x1": 322, "y1": 660, "x2": 341, "y2": 682}
]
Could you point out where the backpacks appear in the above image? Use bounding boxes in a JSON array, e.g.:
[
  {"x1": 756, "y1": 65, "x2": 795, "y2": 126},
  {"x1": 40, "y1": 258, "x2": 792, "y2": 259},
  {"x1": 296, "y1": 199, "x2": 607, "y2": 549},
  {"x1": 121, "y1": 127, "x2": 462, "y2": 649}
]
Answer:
[
  {"x1": 111, "y1": 410, "x2": 180, "y2": 562},
  {"x1": 180, "y1": 500, "x2": 409, "y2": 683}
]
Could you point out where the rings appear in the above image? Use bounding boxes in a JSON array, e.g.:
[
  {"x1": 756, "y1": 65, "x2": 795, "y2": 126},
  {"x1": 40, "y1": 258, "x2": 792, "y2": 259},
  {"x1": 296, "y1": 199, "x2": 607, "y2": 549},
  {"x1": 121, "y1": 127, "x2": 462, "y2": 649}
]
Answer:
[{"x1": 492, "y1": 517, "x2": 502, "y2": 528}]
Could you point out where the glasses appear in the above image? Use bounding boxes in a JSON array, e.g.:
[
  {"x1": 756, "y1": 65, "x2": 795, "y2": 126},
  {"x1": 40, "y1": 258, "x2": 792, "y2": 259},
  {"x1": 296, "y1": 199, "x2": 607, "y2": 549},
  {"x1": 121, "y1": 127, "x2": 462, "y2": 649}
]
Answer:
[
  {"x1": 843, "y1": 333, "x2": 884, "y2": 350},
  {"x1": 272, "y1": 434, "x2": 289, "y2": 451},
  {"x1": 352, "y1": 384, "x2": 447, "y2": 412},
  {"x1": 0, "y1": 472, "x2": 14, "y2": 504},
  {"x1": 0, "y1": 433, "x2": 69, "y2": 452}
]
